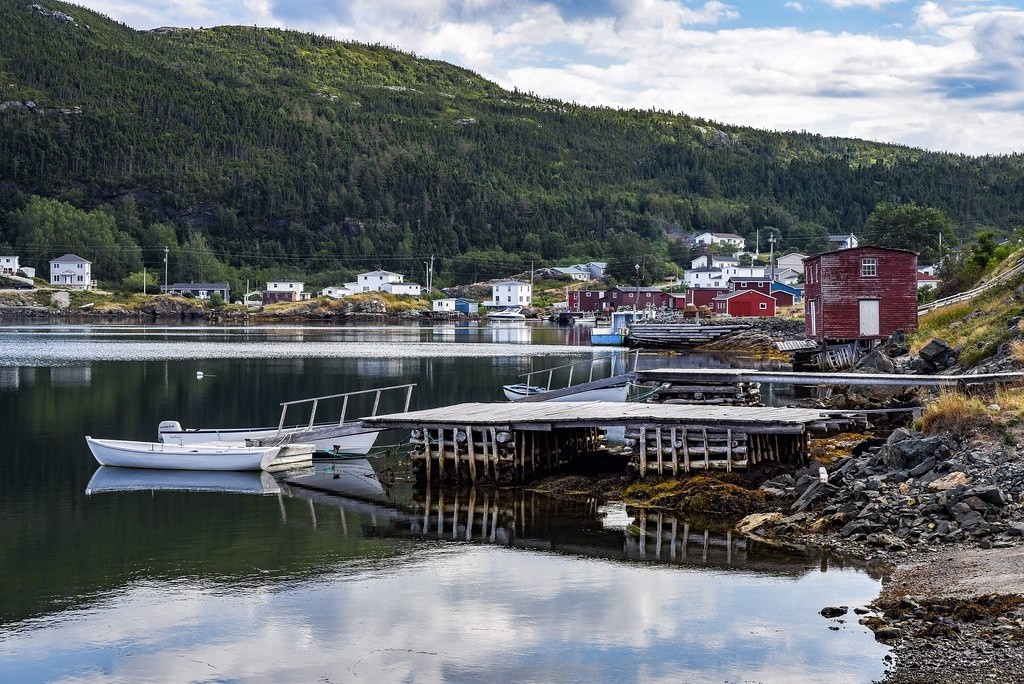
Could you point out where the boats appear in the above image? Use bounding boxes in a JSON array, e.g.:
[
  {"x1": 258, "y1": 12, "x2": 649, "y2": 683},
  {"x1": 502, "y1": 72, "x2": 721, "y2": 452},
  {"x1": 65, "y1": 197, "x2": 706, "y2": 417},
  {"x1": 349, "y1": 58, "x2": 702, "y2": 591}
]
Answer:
[
  {"x1": 286, "y1": 458, "x2": 395, "y2": 516},
  {"x1": 85, "y1": 435, "x2": 317, "y2": 470},
  {"x1": 158, "y1": 420, "x2": 380, "y2": 456},
  {"x1": 85, "y1": 465, "x2": 283, "y2": 496},
  {"x1": 503, "y1": 382, "x2": 549, "y2": 401}
]
